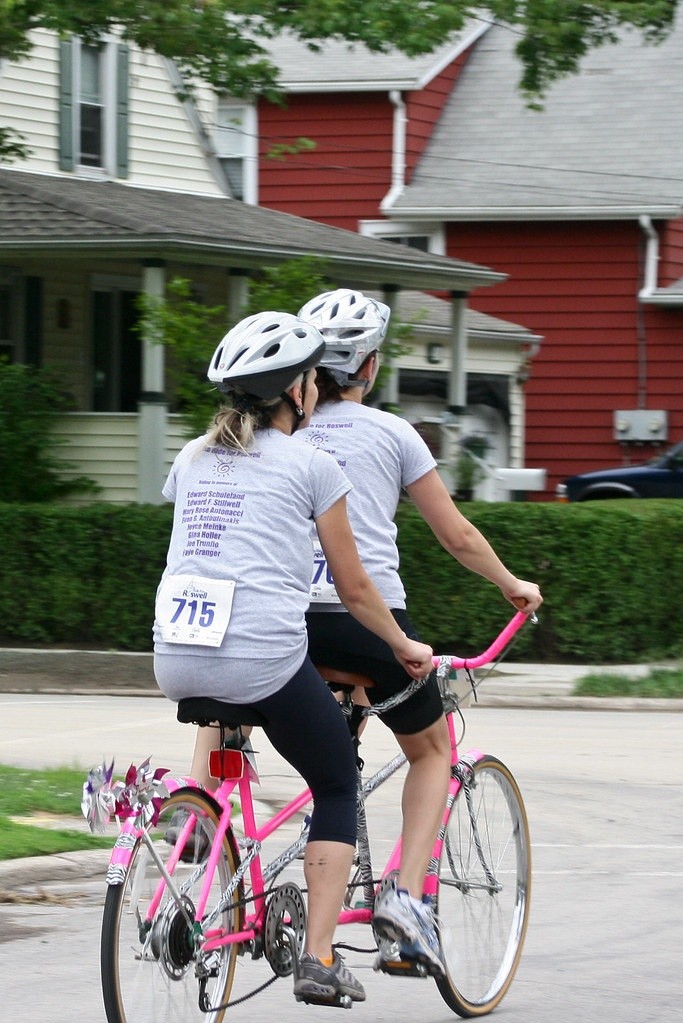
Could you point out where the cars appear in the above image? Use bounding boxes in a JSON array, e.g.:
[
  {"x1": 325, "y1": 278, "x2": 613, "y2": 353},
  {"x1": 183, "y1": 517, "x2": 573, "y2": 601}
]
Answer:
[{"x1": 562, "y1": 438, "x2": 683, "y2": 502}]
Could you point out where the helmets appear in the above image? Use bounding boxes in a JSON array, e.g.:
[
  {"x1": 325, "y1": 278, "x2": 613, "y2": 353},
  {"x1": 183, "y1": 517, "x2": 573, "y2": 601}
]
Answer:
[
  {"x1": 299, "y1": 288, "x2": 394, "y2": 374},
  {"x1": 203, "y1": 310, "x2": 324, "y2": 401}
]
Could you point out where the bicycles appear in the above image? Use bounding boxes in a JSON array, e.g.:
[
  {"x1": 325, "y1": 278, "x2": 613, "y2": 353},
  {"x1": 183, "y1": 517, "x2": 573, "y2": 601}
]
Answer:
[{"x1": 78, "y1": 597, "x2": 534, "y2": 1023}]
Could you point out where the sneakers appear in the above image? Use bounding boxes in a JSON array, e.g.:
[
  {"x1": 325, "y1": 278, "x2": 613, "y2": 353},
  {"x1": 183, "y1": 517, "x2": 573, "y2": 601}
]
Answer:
[
  {"x1": 294, "y1": 948, "x2": 367, "y2": 1000},
  {"x1": 165, "y1": 805, "x2": 212, "y2": 860},
  {"x1": 376, "y1": 889, "x2": 446, "y2": 981}
]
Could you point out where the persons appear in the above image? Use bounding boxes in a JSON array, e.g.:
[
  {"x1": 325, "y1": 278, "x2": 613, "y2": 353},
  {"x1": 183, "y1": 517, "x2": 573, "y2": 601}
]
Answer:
[
  {"x1": 294, "y1": 287, "x2": 543, "y2": 979},
  {"x1": 152, "y1": 310, "x2": 433, "y2": 1002}
]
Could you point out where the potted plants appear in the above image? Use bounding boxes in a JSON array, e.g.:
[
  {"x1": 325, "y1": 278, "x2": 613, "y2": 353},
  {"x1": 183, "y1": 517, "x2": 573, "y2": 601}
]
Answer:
[{"x1": 442, "y1": 444, "x2": 489, "y2": 502}]
